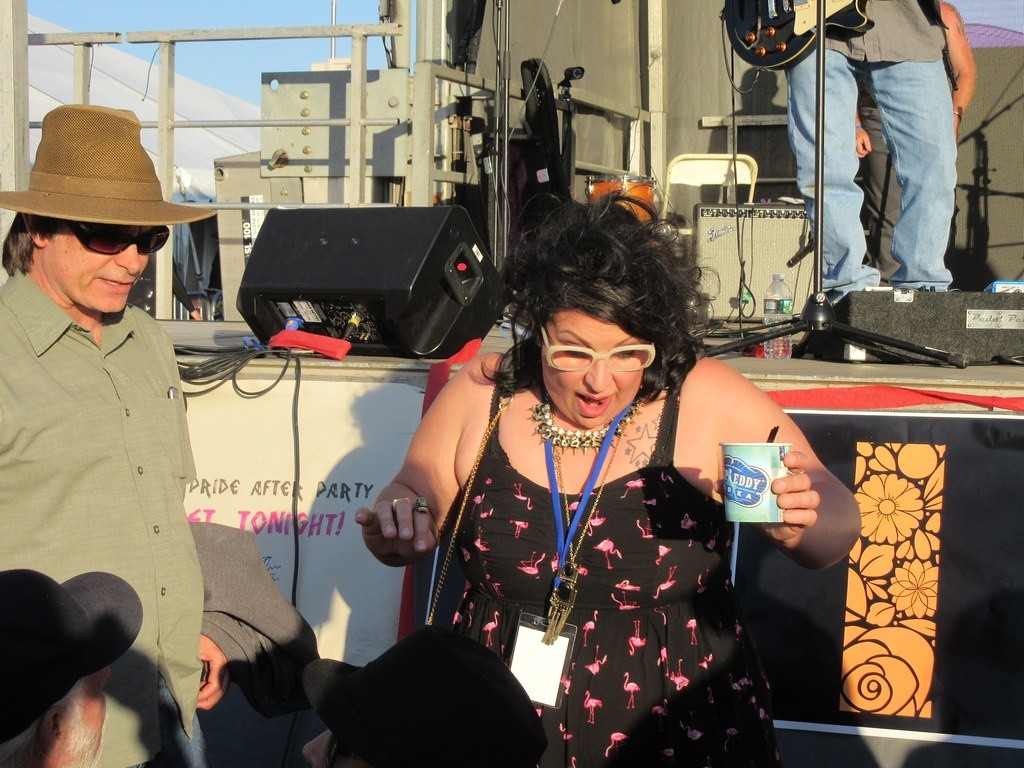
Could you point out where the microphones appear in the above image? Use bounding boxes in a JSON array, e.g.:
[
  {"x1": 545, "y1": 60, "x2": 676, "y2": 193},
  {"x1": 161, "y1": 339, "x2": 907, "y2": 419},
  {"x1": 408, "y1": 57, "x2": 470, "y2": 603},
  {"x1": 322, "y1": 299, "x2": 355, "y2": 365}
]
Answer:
[{"x1": 788, "y1": 244, "x2": 814, "y2": 267}]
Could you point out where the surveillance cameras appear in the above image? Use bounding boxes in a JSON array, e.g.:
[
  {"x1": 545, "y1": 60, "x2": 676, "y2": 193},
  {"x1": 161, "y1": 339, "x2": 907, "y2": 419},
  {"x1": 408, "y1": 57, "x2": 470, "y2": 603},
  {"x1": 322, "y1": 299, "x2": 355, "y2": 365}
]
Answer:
[{"x1": 564, "y1": 67, "x2": 585, "y2": 80}]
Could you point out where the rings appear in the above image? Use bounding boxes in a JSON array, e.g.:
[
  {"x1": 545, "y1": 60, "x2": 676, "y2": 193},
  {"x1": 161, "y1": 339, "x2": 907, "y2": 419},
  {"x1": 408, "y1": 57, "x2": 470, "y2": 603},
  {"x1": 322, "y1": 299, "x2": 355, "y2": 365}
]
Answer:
[
  {"x1": 416, "y1": 497, "x2": 430, "y2": 513},
  {"x1": 391, "y1": 497, "x2": 412, "y2": 512}
]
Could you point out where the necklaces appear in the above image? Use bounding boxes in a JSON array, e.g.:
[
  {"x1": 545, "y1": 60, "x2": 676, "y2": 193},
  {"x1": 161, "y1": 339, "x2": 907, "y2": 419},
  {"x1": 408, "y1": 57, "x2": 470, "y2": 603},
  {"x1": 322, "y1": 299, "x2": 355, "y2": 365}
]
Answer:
[{"x1": 527, "y1": 394, "x2": 644, "y2": 645}]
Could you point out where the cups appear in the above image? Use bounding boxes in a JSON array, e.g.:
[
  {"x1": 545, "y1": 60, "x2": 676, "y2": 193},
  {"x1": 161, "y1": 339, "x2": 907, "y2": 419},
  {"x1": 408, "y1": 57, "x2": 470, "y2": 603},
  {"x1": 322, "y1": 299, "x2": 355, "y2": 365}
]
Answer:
[{"x1": 718, "y1": 441, "x2": 793, "y2": 522}]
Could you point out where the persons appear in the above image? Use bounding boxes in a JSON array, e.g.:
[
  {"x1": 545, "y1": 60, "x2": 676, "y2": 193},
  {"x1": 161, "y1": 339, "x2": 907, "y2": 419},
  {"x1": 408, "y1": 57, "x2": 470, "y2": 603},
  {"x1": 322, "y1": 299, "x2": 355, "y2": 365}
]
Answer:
[
  {"x1": 785, "y1": 0, "x2": 976, "y2": 303},
  {"x1": 355, "y1": 188, "x2": 862, "y2": 767},
  {"x1": 1, "y1": 101, "x2": 230, "y2": 768},
  {"x1": 0, "y1": 556, "x2": 553, "y2": 768}
]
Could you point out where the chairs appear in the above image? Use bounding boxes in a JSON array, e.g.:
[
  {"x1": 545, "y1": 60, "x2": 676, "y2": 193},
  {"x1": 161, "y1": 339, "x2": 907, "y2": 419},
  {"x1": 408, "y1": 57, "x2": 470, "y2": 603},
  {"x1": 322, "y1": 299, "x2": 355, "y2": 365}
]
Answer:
[{"x1": 660, "y1": 152, "x2": 758, "y2": 234}]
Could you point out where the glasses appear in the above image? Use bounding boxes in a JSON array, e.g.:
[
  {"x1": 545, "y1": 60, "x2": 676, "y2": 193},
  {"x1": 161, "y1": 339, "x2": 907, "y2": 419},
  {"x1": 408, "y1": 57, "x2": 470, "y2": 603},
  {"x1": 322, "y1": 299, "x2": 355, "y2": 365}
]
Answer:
[
  {"x1": 326, "y1": 732, "x2": 363, "y2": 768},
  {"x1": 539, "y1": 320, "x2": 655, "y2": 373},
  {"x1": 62, "y1": 220, "x2": 169, "y2": 254}
]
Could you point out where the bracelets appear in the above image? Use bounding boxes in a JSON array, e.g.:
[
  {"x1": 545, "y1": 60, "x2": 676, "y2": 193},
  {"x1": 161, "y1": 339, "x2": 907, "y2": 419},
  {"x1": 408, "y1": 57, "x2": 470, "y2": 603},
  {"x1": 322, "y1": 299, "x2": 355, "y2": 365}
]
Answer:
[
  {"x1": 953, "y1": 112, "x2": 961, "y2": 123},
  {"x1": 856, "y1": 125, "x2": 862, "y2": 130}
]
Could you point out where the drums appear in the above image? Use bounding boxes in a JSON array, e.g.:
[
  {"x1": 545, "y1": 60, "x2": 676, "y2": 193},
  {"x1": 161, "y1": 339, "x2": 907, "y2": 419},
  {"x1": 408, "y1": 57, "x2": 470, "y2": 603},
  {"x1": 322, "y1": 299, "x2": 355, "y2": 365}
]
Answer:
[{"x1": 585, "y1": 173, "x2": 655, "y2": 225}]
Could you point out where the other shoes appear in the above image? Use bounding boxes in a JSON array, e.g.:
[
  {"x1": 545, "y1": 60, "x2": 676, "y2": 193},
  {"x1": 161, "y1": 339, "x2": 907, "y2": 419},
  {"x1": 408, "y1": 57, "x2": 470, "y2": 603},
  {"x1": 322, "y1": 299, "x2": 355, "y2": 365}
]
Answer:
[{"x1": 796, "y1": 304, "x2": 849, "y2": 358}]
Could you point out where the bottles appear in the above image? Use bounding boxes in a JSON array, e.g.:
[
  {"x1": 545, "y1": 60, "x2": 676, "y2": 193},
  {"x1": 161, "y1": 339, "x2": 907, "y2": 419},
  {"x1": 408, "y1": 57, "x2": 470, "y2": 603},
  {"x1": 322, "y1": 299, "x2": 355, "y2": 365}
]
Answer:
[{"x1": 764, "y1": 273, "x2": 793, "y2": 359}]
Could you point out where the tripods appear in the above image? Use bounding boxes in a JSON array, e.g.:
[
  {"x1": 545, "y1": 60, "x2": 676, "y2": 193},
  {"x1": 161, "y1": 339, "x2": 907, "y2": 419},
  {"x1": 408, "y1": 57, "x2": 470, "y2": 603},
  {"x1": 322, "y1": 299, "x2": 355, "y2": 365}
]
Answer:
[{"x1": 704, "y1": 0, "x2": 970, "y2": 370}]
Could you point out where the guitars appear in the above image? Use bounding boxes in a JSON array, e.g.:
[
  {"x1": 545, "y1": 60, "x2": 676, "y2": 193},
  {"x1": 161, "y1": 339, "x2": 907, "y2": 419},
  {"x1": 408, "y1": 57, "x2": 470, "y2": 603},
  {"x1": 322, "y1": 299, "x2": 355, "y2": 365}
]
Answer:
[{"x1": 723, "y1": 0, "x2": 876, "y2": 70}]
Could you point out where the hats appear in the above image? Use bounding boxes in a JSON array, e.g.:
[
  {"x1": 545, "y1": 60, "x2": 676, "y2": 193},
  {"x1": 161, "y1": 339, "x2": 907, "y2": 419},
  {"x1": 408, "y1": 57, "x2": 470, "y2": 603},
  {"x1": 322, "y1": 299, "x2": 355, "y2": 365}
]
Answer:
[
  {"x1": 302, "y1": 625, "x2": 548, "y2": 768},
  {"x1": 0, "y1": 103, "x2": 217, "y2": 225},
  {"x1": 0, "y1": 568, "x2": 143, "y2": 746}
]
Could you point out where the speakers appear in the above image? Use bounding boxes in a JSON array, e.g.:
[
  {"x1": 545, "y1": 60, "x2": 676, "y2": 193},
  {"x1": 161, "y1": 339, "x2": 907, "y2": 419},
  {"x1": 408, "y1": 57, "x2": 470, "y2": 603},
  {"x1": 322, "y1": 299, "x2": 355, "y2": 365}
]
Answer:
[
  {"x1": 688, "y1": 204, "x2": 815, "y2": 323},
  {"x1": 236, "y1": 205, "x2": 505, "y2": 359}
]
